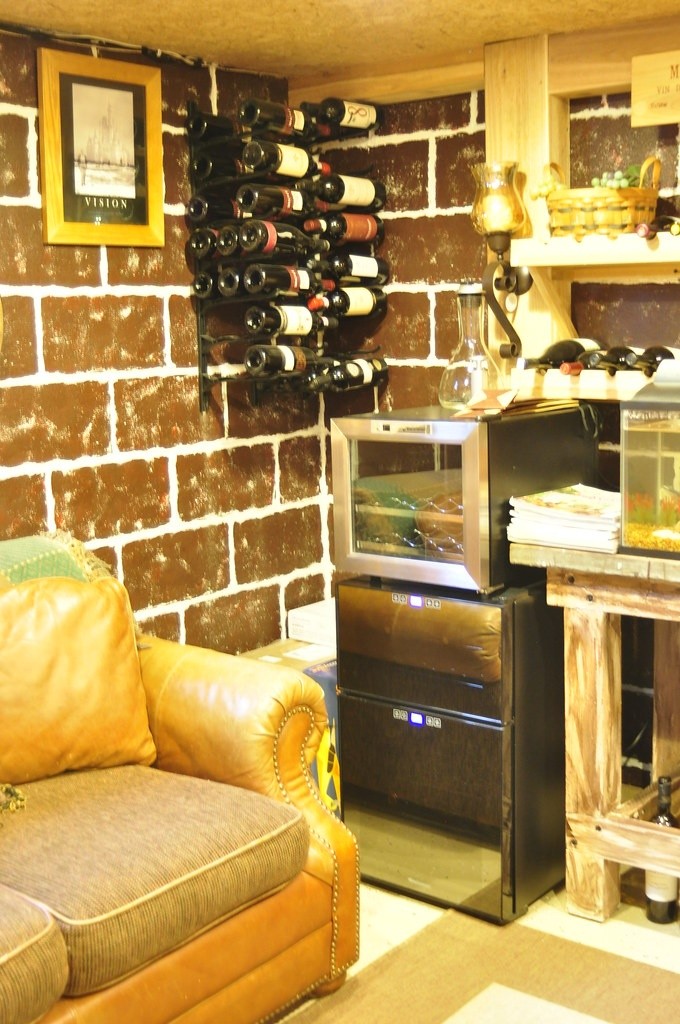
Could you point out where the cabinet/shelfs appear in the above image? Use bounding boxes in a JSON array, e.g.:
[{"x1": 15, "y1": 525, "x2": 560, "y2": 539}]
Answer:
[{"x1": 186, "y1": 96, "x2": 395, "y2": 412}]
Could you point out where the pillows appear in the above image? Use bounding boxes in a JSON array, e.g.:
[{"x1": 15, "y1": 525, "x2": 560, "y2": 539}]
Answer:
[{"x1": 3, "y1": 575, "x2": 158, "y2": 786}]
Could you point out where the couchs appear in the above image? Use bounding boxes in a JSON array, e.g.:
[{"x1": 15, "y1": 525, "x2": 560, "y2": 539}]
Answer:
[{"x1": 2, "y1": 528, "x2": 363, "y2": 1022}]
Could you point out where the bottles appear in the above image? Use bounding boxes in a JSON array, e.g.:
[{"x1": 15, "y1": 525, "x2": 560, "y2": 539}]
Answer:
[
  {"x1": 668, "y1": 219, "x2": 679, "y2": 238},
  {"x1": 515, "y1": 337, "x2": 606, "y2": 369},
  {"x1": 589, "y1": 345, "x2": 647, "y2": 371},
  {"x1": 561, "y1": 347, "x2": 607, "y2": 376},
  {"x1": 436, "y1": 283, "x2": 506, "y2": 411},
  {"x1": 627, "y1": 345, "x2": 679, "y2": 373},
  {"x1": 183, "y1": 96, "x2": 390, "y2": 396},
  {"x1": 636, "y1": 214, "x2": 679, "y2": 239},
  {"x1": 645, "y1": 777, "x2": 679, "y2": 925}
]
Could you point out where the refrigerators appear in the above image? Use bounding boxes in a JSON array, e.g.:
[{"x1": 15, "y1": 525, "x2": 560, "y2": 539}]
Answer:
[
  {"x1": 323, "y1": 579, "x2": 646, "y2": 924},
  {"x1": 330, "y1": 405, "x2": 598, "y2": 599}
]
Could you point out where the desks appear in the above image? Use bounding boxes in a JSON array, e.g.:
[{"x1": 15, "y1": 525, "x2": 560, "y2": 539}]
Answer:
[{"x1": 506, "y1": 550, "x2": 680, "y2": 928}]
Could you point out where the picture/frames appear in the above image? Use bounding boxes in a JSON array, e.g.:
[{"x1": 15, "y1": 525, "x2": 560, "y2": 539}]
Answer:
[{"x1": 38, "y1": 46, "x2": 167, "y2": 248}]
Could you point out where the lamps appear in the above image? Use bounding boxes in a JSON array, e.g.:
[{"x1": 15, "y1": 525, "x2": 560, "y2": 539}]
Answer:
[{"x1": 465, "y1": 153, "x2": 535, "y2": 359}]
